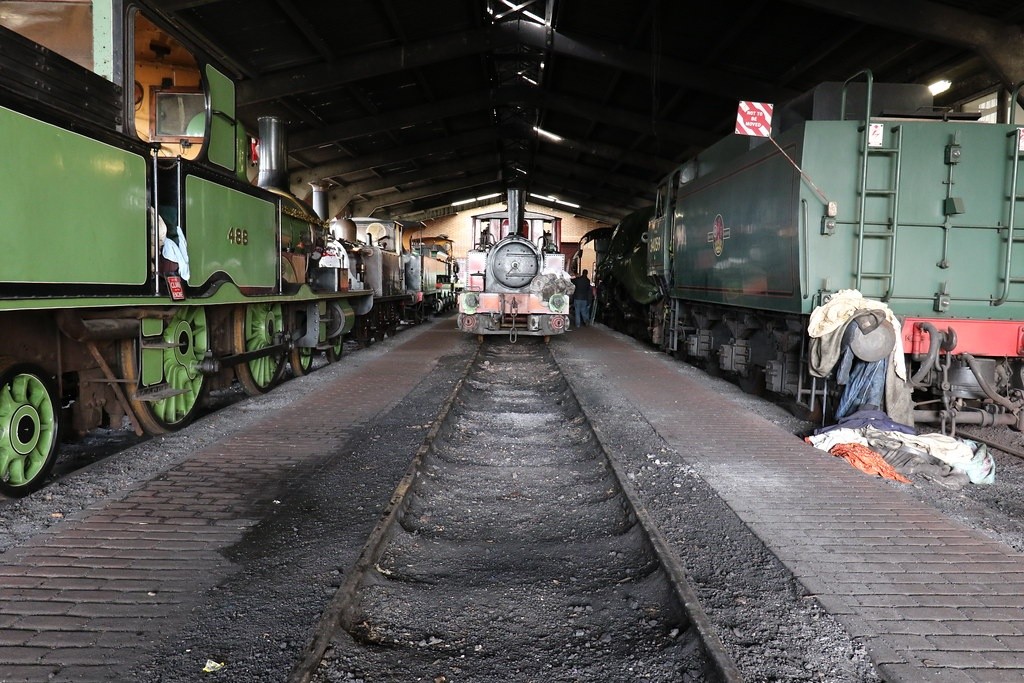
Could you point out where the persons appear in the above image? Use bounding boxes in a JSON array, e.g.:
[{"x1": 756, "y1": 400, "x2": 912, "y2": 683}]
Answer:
[{"x1": 569, "y1": 269, "x2": 592, "y2": 329}]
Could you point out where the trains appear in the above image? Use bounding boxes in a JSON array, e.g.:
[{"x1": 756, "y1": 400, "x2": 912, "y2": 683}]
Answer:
[
  {"x1": 568, "y1": 68, "x2": 1024, "y2": 426},
  {"x1": 454, "y1": 209, "x2": 576, "y2": 344},
  {"x1": 0, "y1": 0, "x2": 458, "y2": 499}
]
